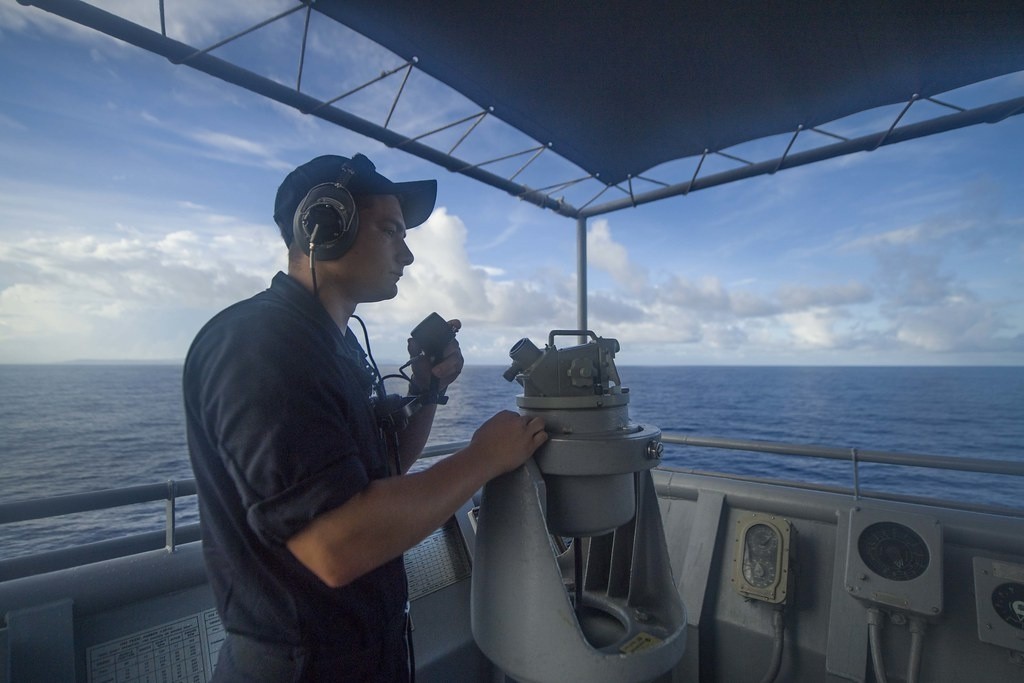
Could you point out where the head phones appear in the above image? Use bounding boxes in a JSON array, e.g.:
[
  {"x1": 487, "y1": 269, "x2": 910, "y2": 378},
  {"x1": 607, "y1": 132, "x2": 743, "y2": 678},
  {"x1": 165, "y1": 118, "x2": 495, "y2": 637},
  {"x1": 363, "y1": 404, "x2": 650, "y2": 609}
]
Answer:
[{"x1": 293, "y1": 153, "x2": 376, "y2": 260}]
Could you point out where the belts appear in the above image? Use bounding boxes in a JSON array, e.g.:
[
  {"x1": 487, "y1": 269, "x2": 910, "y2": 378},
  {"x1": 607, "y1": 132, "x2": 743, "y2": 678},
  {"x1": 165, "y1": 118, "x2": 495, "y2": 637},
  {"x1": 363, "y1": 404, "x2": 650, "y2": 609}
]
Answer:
[{"x1": 228, "y1": 622, "x2": 415, "y2": 683}]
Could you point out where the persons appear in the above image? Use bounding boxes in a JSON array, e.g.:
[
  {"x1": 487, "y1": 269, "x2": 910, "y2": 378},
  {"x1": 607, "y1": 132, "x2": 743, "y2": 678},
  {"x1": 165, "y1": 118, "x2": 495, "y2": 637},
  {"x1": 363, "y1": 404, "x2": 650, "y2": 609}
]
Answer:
[{"x1": 181, "y1": 153, "x2": 549, "y2": 683}]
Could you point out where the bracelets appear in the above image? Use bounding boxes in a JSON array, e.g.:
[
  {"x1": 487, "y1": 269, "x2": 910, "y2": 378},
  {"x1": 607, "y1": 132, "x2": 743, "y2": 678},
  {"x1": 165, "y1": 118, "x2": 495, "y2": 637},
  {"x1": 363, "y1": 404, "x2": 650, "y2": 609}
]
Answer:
[{"x1": 409, "y1": 378, "x2": 449, "y2": 406}]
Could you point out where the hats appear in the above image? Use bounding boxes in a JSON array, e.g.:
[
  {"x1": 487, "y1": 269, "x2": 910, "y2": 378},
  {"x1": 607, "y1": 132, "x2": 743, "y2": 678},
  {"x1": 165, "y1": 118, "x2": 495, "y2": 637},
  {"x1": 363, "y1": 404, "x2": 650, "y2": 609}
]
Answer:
[{"x1": 275, "y1": 155, "x2": 437, "y2": 247}]
